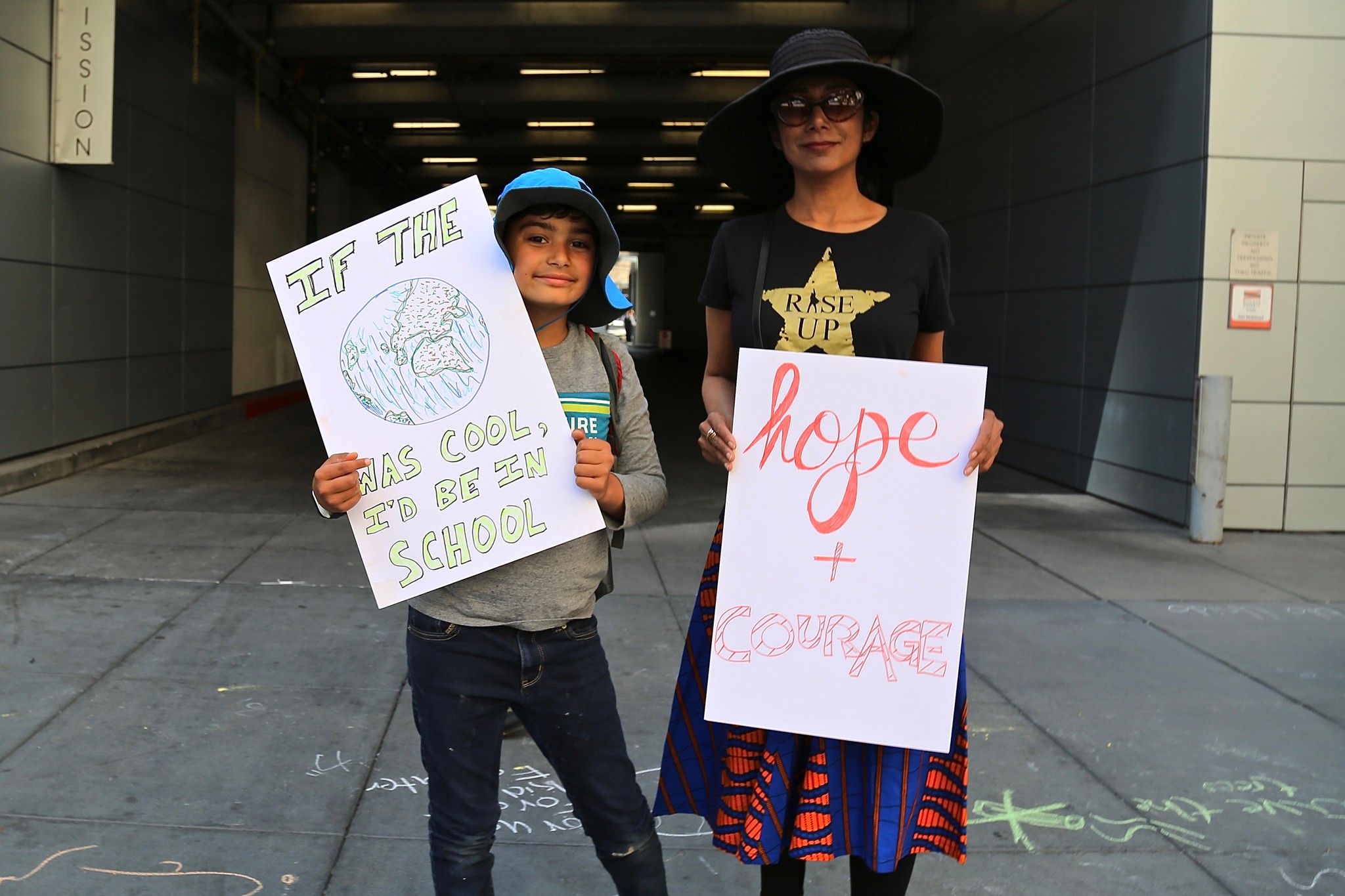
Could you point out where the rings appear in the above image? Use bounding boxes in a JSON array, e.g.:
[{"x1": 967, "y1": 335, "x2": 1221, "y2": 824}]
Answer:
[{"x1": 706, "y1": 428, "x2": 716, "y2": 443}]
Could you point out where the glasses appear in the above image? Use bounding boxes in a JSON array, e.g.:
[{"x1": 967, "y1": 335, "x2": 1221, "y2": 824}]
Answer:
[{"x1": 773, "y1": 81, "x2": 865, "y2": 127}]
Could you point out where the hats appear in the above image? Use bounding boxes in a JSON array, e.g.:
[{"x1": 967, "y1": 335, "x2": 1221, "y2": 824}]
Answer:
[
  {"x1": 695, "y1": 28, "x2": 944, "y2": 199},
  {"x1": 492, "y1": 168, "x2": 635, "y2": 327}
]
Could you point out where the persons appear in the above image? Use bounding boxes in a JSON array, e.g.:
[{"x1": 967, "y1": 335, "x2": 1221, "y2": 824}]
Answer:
[
  {"x1": 651, "y1": 29, "x2": 1005, "y2": 896},
  {"x1": 312, "y1": 169, "x2": 668, "y2": 896}
]
